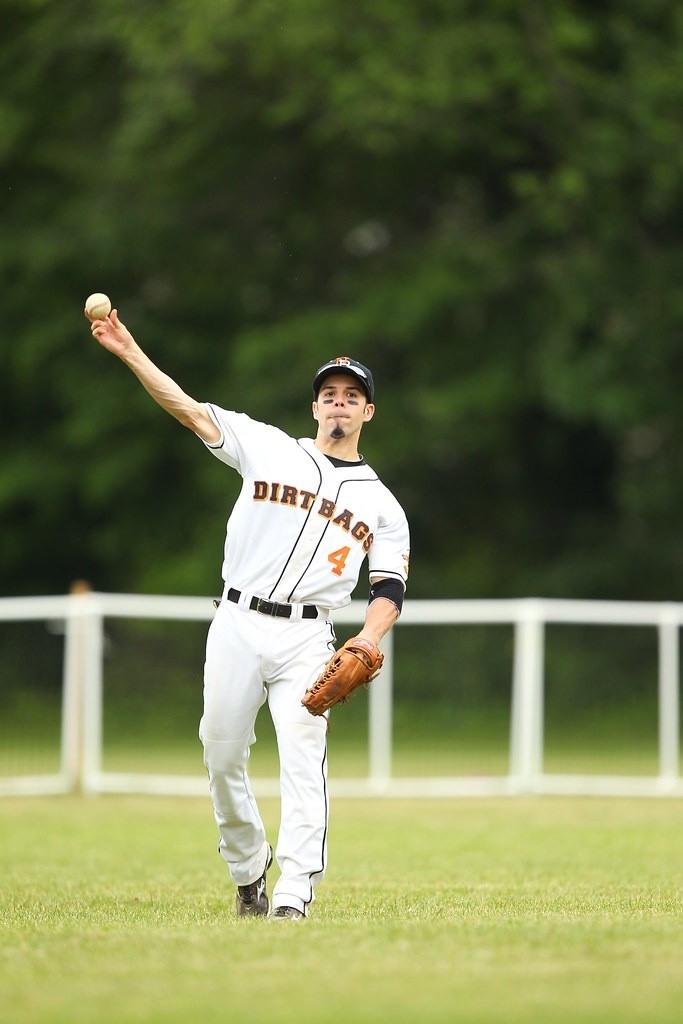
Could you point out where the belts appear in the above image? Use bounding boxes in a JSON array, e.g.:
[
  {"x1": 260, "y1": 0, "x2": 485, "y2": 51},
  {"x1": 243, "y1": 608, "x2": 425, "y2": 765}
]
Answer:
[{"x1": 228, "y1": 589, "x2": 317, "y2": 618}]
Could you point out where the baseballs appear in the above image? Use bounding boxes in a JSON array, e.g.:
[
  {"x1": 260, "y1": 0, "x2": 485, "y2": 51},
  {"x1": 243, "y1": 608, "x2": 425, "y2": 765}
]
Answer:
[{"x1": 86, "y1": 292, "x2": 112, "y2": 320}]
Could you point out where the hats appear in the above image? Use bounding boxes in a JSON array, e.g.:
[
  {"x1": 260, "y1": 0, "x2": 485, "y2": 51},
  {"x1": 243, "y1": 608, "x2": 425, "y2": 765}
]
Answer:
[{"x1": 312, "y1": 356, "x2": 374, "y2": 404}]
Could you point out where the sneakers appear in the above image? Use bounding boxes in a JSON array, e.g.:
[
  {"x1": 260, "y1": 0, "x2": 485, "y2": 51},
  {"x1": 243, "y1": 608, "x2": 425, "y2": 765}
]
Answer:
[
  {"x1": 269, "y1": 906, "x2": 306, "y2": 921},
  {"x1": 236, "y1": 846, "x2": 274, "y2": 916}
]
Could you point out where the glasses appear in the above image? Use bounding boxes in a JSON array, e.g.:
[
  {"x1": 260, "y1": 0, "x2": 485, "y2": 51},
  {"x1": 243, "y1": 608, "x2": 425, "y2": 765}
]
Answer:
[{"x1": 317, "y1": 364, "x2": 372, "y2": 391}]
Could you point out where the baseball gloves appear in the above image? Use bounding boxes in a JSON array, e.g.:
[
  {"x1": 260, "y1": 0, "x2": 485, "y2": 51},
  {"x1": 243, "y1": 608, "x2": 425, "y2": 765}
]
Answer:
[{"x1": 301, "y1": 637, "x2": 384, "y2": 717}]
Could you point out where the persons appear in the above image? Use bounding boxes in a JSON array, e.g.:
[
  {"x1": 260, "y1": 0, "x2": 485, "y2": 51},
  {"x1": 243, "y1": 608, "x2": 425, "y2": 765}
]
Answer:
[{"x1": 85, "y1": 290, "x2": 411, "y2": 920}]
